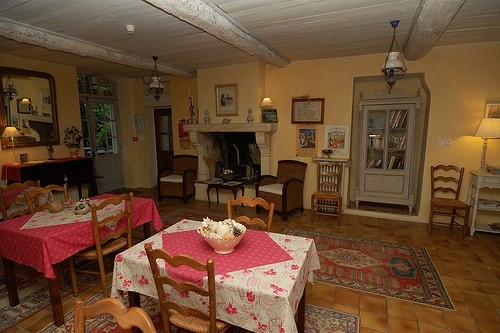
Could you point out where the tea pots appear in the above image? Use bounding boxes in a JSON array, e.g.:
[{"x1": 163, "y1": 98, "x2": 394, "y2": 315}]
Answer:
[{"x1": 47, "y1": 198, "x2": 64, "y2": 213}]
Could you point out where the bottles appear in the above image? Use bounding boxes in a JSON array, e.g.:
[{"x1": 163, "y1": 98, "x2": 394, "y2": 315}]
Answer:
[
  {"x1": 247, "y1": 109, "x2": 254, "y2": 124},
  {"x1": 48, "y1": 140, "x2": 54, "y2": 159},
  {"x1": 204, "y1": 109, "x2": 210, "y2": 124}
]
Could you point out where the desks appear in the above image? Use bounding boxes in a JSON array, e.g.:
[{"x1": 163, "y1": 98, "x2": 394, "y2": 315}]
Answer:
[
  {"x1": 207, "y1": 178, "x2": 244, "y2": 211},
  {"x1": 0, "y1": 158, "x2": 97, "y2": 199},
  {"x1": 0, "y1": 194, "x2": 162, "y2": 327},
  {"x1": 98, "y1": 216, "x2": 320, "y2": 333}
]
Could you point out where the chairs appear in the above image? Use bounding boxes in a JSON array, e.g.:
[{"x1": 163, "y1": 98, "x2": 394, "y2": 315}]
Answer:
[
  {"x1": 157, "y1": 154, "x2": 198, "y2": 204},
  {"x1": 256, "y1": 160, "x2": 308, "y2": 220},
  {"x1": 429, "y1": 165, "x2": 470, "y2": 236},
  {"x1": 0, "y1": 180, "x2": 274, "y2": 333},
  {"x1": 310, "y1": 160, "x2": 344, "y2": 224}
]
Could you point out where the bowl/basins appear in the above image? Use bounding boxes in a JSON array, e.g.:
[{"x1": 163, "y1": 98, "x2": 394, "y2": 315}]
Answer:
[
  {"x1": 222, "y1": 175, "x2": 233, "y2": 180},
  {"x1": 197, "y1": 220, "x2": 247, "y2": 254}
]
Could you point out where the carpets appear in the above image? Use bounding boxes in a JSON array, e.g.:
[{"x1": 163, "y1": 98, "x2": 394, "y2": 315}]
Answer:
[
  {"x1": 0, "y1": 235, "x2": 144, "y2": 332},
  {"x1": 284, "y1": 228, "x2": 457, "y2": 312},
  {"x1": 33, "y1": 278, "x2": 359, "y2": 333}
]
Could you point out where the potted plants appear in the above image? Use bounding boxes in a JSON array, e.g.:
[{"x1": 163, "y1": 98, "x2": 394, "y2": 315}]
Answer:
[{"x1": 62, "y1": 127, "x2": 84, "y2": 159}]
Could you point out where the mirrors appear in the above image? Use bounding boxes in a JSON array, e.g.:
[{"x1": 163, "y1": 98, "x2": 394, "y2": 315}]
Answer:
[{"x1": 0, "y1": 66, "x2": 58, "y2": 150}]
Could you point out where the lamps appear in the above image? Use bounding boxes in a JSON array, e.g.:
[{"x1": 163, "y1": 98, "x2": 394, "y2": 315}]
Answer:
[
  {"x1": 474, "y1": 118, "x2": 500, "y2": 174},
  {"x1": 261, "y1": 97, "x2": 273, "y2": 123},
  {"x1": 149, "y1": 56, "x2": 165, "y2": 91},
  {"x1": 0, "y1": 127, "x2": 22, "y2": 163},
  {"x1": 3, "y1": 74, "x2": 18, "y2": 102},
  {"x1": 382, "y1": 20, "x2": 408, "y2": 79},
  {"x1": 22, "y1": 98, "x2": 31, "y2": 114}
]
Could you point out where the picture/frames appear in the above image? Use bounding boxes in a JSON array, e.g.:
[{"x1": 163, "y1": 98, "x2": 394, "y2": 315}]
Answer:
[
  {"x1": 483, "y1": 103, "x2": 500, "y2": 139},
  {"x1": 17, "y1": 98, "x2": 31, "y2": 114},
  {"x1": 291, "y1": 97, "x2": 325, "y2": 124},
  {"x1": 214, "y1": 83, "x2": 238, "y2": 117}
]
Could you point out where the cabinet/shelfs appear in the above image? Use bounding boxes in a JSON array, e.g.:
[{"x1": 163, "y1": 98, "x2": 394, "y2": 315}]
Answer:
[
  {"x1": 355, "y1": 78, "x2": 421, "y2": 213},
  {"x1": 465, "y1": 172, "x2": 500, "y2": 236}
]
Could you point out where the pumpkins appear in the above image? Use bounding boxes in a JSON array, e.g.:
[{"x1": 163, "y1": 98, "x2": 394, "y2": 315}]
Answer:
[{"x1": 48, "y1": 200, "x2": 64, "y2": 213}]
